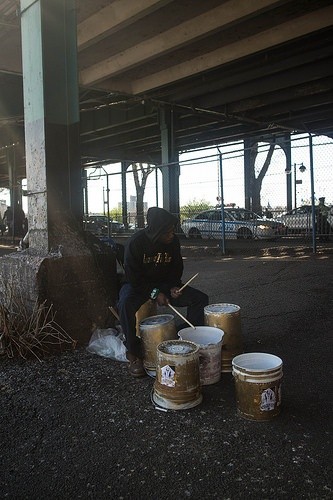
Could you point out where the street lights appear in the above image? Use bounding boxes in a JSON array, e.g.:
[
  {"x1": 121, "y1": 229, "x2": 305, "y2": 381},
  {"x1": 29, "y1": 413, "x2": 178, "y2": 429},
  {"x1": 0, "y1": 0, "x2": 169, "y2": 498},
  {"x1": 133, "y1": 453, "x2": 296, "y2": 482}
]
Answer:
[{"x1": 285, "y1": 163, "x2": 307, "y2": 216}]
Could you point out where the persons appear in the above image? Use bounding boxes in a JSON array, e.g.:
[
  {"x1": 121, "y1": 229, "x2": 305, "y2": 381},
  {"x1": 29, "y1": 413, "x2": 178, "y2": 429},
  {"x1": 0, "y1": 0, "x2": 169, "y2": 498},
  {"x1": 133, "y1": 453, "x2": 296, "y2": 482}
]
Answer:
[
  {"x1": 2, "y1": 206, "x2": 10, "y2": 232},
  {"x1": 316, "y1": 197, "x2": 329, "y2": 242},
  {"x1": 117, "y1": 207, "x2": 209, "y2": 376}
]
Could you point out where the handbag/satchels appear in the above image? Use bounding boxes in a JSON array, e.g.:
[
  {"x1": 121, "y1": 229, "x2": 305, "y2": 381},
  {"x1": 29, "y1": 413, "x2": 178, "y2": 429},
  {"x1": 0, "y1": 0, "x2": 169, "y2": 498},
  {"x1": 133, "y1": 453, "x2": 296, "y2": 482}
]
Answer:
[{"x1": 85, "y1": 328, "x2": 130, "y2": 362}]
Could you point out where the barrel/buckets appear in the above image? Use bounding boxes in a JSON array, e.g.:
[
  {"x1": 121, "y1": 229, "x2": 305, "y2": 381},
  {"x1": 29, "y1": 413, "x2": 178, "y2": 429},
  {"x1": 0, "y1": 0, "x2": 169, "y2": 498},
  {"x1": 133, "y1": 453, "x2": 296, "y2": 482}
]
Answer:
[
  {"x1": 150, "y1": 340, "x2": 202, "y2": 412},
  {"x1": 140, "y1": 314, "x2": 177, "y2": 378},
  {"x1": 178, "y1": 325, "x2": 225, "y2": 385},
  {"x1": 135, "y1": 300, "x2": 157, "y2": 337},
  {"x1": 232, "y1": 352, "x2": 284, "y2": 423},
  {"x1": 204, "y1": 302, "x2": 243, "y2": 373}
]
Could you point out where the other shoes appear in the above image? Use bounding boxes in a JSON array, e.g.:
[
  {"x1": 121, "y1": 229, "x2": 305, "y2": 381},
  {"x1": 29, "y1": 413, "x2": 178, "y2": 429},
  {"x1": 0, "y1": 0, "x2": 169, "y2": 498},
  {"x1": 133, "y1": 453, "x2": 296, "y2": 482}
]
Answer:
[{"x1": 126, "y1": 353, "x2": 146, "y2": 377}]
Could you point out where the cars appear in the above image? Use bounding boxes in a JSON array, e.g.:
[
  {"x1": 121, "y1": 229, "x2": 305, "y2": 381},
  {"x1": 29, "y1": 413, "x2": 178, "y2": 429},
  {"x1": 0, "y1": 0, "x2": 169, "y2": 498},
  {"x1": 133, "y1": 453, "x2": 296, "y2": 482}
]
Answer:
[
  {"x1": 270, "y1": 204, "x2": 333, "y2": 233},
  {"x1": 181, "y1": 206, "x2": 286, "y2": 240},
  {"x1": 82, "y1": 216, "x2": 149, "y2": 232}
]
